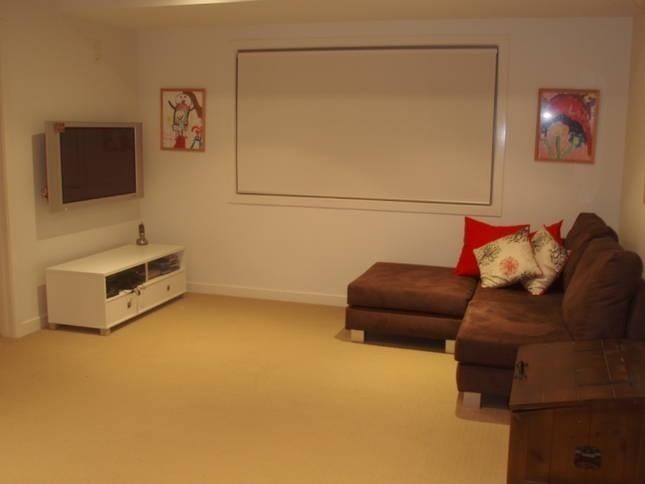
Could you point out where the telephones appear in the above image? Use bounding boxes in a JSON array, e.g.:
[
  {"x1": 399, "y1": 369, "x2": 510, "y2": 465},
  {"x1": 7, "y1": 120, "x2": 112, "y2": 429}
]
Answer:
[{"x1": 136, "y1": 222, "x2": 148, "y2": 245}]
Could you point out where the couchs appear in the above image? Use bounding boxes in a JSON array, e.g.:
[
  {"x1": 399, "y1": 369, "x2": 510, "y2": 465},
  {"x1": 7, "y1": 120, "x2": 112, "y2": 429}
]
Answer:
[{"x1": 454, "y1": 213, "x2": 642, "y2": 408}]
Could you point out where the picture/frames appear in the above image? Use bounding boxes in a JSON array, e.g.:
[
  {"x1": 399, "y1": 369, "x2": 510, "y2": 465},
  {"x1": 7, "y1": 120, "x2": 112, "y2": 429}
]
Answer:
[
  {"x1": 160, "y1": 88, "x2": 206, "y2": 152},
  {"x1": 534, "y1": 88, "x2": 600, "y2": 164}
]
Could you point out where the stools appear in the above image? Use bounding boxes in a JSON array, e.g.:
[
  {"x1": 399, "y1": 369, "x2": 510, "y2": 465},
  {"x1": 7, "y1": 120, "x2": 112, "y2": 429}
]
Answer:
[{"x1": 345, "y1": 261, "x2": 475, "y2": 353}]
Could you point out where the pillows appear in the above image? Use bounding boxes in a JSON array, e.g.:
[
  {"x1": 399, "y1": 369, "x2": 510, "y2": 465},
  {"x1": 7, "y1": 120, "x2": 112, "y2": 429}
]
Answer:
[{"x1": 454, "y1": 216, "x2": 573, "y2": 297}]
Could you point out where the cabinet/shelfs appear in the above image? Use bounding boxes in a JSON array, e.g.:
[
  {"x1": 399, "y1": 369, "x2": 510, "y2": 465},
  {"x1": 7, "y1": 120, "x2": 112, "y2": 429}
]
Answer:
[{"x1": 46, "y1": 243, "x2": 185, "y2": 335}]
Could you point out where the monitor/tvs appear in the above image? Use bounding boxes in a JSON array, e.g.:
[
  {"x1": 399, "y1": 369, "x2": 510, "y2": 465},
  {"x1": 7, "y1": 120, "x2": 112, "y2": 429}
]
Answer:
[{"x1": 44, "y1": 119, "x2": 144, "y2": 219}]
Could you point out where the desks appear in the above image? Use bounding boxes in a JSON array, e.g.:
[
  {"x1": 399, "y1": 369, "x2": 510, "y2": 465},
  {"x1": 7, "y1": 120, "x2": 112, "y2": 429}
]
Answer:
[{"x1": 507, "y1": 338, "x2": 645, "y2": 484}]
